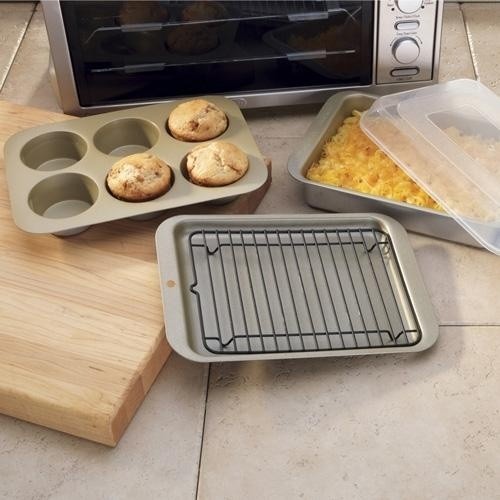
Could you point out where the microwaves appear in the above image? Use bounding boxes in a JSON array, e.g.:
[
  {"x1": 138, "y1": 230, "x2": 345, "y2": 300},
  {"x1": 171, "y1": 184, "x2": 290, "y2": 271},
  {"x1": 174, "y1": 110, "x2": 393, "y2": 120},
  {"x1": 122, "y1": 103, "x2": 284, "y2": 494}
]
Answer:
[{"x1": 38, "y1": 0, "x2": 445, "y2": 120}]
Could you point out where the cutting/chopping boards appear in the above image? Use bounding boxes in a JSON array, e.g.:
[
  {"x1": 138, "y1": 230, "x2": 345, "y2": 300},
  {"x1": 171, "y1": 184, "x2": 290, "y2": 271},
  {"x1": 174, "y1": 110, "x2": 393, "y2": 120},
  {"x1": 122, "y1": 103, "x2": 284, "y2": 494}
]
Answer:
[{"x1": 0, "y1": 98, "x2": 274, "y2": 450}]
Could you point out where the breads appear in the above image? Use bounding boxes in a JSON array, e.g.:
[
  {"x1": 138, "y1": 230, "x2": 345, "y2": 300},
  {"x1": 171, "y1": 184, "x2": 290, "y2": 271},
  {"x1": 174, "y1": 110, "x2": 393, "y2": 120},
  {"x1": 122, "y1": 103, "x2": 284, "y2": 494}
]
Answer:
[
  {"x1": 186, "y1": 139, "x2": 249, "y2": 187},
  {"x1": 106, "y1": 152, "x2": 172, "y2": 201},
  {"x1": 167, "y1": 98, "x2": 228, "y2": 141}
]
